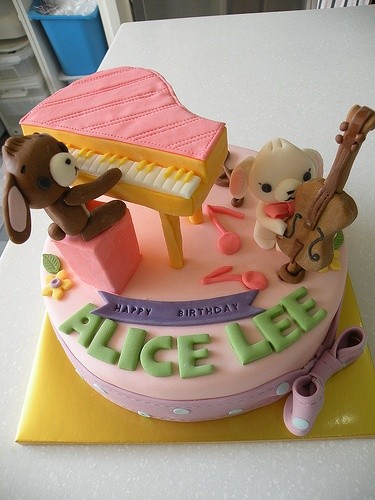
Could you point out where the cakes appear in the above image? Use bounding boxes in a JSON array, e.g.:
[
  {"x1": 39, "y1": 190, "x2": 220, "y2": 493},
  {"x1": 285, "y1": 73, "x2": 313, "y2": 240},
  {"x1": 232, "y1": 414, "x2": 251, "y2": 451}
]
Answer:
[{"x1": 0, "y1": 64, "x2": 375, "y2": 444}]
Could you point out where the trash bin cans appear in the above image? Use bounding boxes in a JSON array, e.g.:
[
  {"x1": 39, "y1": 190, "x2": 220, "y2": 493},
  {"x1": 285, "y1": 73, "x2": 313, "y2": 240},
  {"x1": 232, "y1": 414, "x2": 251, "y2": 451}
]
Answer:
[{"x1": 28, "y1": 0, "x2": 109, "y2": 75}]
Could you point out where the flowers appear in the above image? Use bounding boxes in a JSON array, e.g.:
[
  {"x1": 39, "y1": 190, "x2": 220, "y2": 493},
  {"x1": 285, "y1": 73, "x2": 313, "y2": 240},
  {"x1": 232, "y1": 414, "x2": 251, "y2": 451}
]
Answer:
[{"x1": 41, "y1": 270, "x2": 73, "y2": 301}]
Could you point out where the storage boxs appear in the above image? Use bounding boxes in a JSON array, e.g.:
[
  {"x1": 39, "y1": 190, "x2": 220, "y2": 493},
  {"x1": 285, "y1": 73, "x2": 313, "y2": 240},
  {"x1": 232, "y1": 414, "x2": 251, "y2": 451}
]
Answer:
[
  {"x1": 28, "y1": 0, "x2": 108, "y2": 75},
  {"x1": 0, "y1": 42, "x2": 52, "y2": 136}
]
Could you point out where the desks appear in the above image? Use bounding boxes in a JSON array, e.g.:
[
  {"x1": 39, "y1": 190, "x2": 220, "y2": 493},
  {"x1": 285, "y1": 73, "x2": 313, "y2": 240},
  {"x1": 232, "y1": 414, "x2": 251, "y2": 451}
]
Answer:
[{"x1": 1, "y1": 4, "x2": 375, "y2": 500}]
